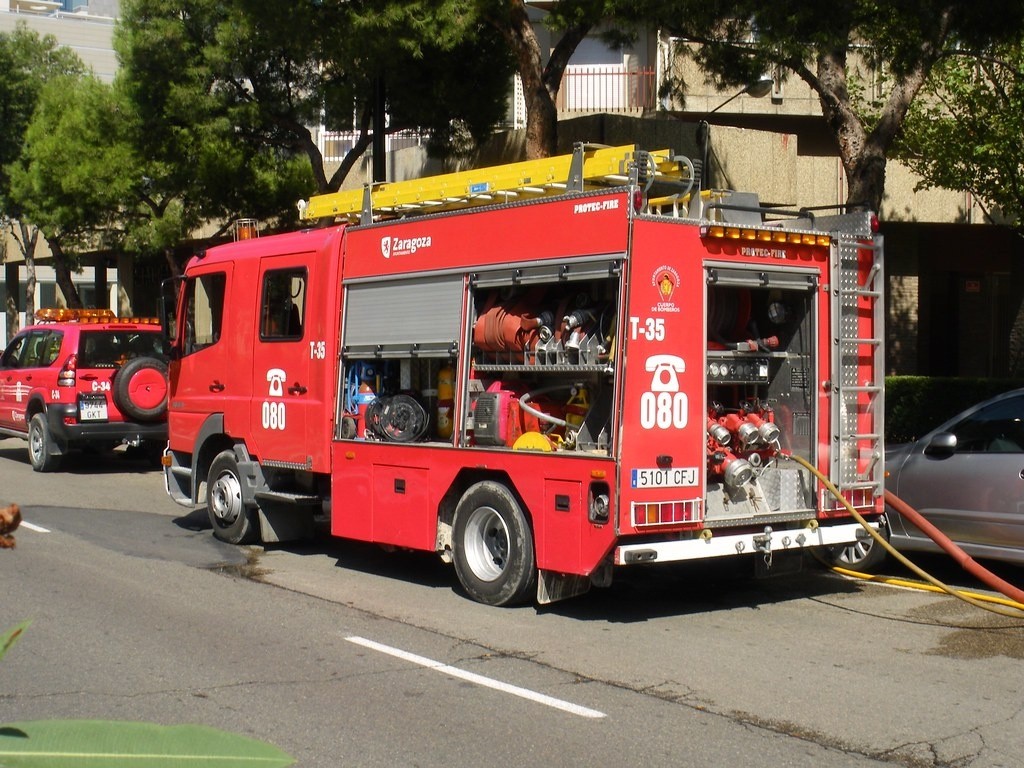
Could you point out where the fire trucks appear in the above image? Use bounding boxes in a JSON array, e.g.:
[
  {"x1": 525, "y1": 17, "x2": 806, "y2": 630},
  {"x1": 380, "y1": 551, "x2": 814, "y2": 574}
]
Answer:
[{"x1": 157, "y1": 141, "x2": 885, "y2": 608}]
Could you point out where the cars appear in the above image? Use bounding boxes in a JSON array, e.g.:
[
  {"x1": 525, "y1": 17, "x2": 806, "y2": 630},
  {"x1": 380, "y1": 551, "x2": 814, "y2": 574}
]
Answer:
[{"x1": 816, "y1": 387, "x2": 1024, "y2": 573}]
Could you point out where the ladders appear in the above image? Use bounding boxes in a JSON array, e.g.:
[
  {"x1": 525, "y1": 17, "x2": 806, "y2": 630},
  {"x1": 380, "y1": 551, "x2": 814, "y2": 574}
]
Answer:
[{"x1": 298, "y1": 144, "x2": 692, "y2": 222}]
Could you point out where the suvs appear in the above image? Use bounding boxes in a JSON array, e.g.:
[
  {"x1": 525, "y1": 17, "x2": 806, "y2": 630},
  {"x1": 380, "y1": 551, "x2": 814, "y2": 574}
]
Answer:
[{"x1": 0, "y1": 306, "x2": 171, "y2": 473}]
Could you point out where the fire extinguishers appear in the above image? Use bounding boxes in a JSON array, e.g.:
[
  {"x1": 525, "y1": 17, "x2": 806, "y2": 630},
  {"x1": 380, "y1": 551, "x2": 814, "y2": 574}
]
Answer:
[{"x1": 436, "y1": 359, "x2": 456, "y2": 438}]
